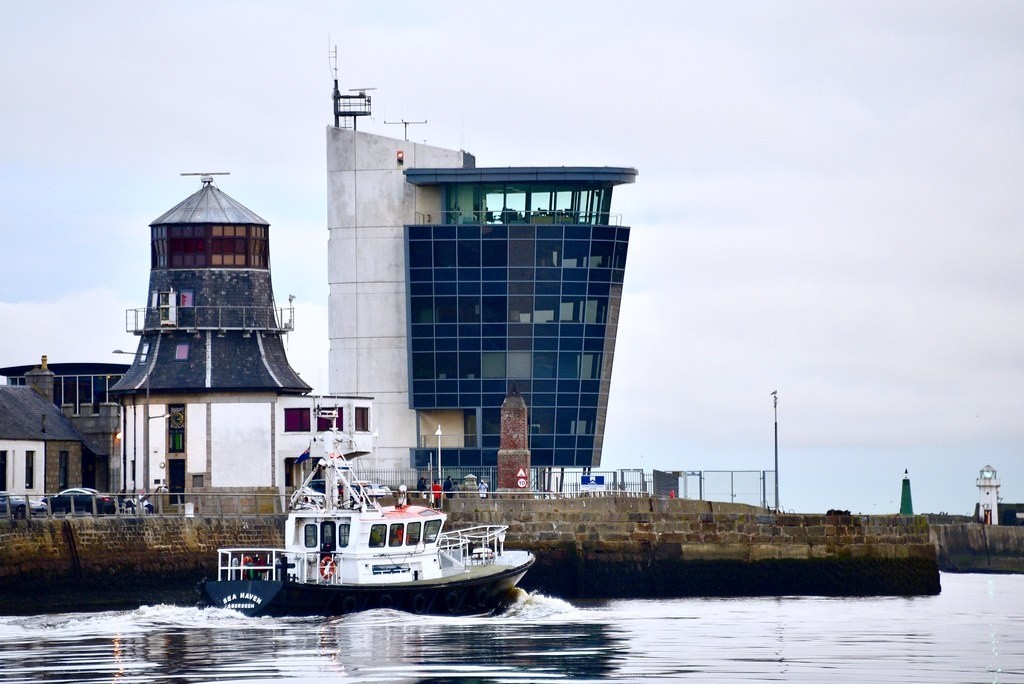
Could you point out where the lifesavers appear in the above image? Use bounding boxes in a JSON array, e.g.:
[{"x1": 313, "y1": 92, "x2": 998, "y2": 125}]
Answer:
[{"x1": 319, "y1": 556, "x2": 335, "y2": 580}]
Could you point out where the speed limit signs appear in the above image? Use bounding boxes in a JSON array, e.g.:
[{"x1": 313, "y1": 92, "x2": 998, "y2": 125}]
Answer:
[{"x1": 518, "y1": 478, "x2": 527, "y2": 488}]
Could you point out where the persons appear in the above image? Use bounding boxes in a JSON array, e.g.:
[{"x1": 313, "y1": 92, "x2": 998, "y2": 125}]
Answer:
[
  {"x1": 450, "y1": 480, "x2": 462, "y2": 498},
  {"x1": 116, "y1": 490, "x2": 136, "y2": 514},
  {"x1": 478, "y1": 479, "x2": 490, "y2": 498},
  {"x1": 444, "y1": 475, "x2": 453, "y2": 499},
  {"x1": 416, "y1": 477, "x2": 427, "y2": 498},
  {"x1": 139, "y1": 490, "x2": 156, "y2": 517},
  {"x1": 395, "y1": 525, "x2": 405, "y2": 544},
  {"x1": 431, "y1": 480, "x2": 441, "y2": 509}
]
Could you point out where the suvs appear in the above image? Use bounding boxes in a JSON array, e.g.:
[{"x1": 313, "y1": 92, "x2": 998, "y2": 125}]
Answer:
[{"x1": 301, "y1": 481, "x2": 392, "y2": 497}]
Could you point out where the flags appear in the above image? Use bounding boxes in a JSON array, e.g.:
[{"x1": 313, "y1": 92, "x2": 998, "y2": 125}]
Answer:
[{"x1": 295, "y1": 443, "x2": 310, "y2": 464}]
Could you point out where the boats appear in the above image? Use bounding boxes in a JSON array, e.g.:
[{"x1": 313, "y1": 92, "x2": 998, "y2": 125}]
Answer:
[{"x1": 197, "y1": 392, "x2": 537, "y2": 619}]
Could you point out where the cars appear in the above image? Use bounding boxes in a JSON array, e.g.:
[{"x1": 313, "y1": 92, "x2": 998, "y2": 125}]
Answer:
[
  {"x1": 42, "y1": 486, "x2": 115, "y2": 515},
  {"x1": 0, "y1": 490, "x2": 47, "y2": 517}
]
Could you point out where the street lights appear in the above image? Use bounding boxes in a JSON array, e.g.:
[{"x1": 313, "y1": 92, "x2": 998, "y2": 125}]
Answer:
[
  {"x1": 435, "y1": 423, "x2": 442, "y2": 488},
  {"x1": 142, "y1": 412, "x2": 171, "y2": 507},
  {"x1": 112, "y1": 350, "x2": 150, "y2": 496},
  {"x1": 770, "y1": 389, "x2": 782, "y2": 514}
]
